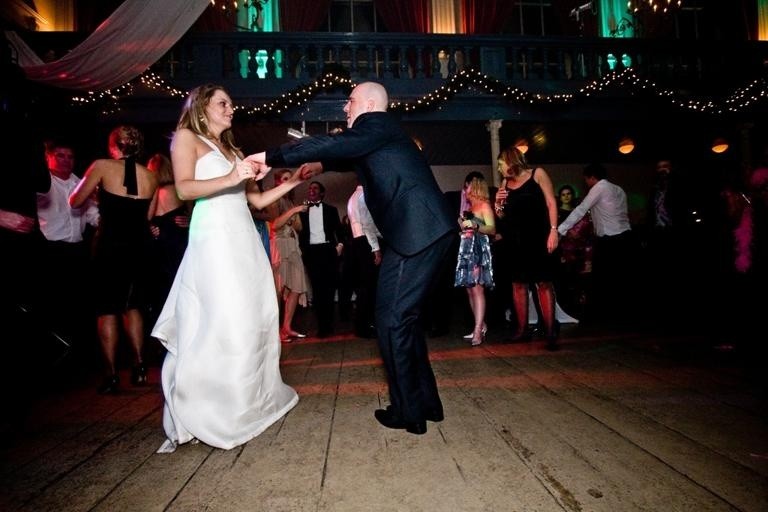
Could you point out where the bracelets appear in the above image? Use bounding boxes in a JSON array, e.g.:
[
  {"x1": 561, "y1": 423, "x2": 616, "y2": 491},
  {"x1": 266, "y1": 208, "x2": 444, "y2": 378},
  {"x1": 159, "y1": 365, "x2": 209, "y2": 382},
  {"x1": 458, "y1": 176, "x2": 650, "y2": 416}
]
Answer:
[
  {"x1": 474, "y1": 224, "x2": 480, "y2": 232},
  {"x1": 550, "y1": 226, "x2": 558, "y2": 230}
]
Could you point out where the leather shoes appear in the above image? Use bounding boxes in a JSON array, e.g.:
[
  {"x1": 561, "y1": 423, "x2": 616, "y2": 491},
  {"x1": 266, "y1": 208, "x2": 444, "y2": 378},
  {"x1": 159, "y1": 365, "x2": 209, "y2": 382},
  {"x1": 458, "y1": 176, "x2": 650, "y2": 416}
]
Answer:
[
  {"x1": 280, "y1": 334, "x2": 293, "y2": 343},
  {"x1": 388, "y1": 404, "x2": 445, "y2": 422},
  {"x1": 289, "y1": 329, "x2": 307, "y2": 338},
  {"x1": 374, "y1": 408, "x2": 427, "y2": 434}
]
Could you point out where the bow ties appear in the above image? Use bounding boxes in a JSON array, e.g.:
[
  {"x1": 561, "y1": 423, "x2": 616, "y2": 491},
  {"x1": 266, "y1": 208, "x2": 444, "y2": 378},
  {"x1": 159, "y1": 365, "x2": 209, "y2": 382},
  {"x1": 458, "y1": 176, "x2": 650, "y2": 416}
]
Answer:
[{"x1": 309, "y1": 202, "x2": 320, "y2": 207}]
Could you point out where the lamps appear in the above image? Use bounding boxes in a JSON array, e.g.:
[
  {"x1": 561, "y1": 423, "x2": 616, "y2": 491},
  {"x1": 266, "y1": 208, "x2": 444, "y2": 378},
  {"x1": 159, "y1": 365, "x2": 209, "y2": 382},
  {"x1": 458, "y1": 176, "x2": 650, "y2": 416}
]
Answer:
[
  {"x1": 712, "y1": 137, "x2": 730, "y2": 154},
  {"x1": 611, "y1": 0, "x2": 684, "y2": 36},
  {"x1": 514, "y1": 135, "x2": 529, "y2": 154},
  {"x1": 209, "y1": 0, "x2": 268, "y2": 33},
  {"x1": 619, "y1": 137, "x2": 635, "y2": 155}
]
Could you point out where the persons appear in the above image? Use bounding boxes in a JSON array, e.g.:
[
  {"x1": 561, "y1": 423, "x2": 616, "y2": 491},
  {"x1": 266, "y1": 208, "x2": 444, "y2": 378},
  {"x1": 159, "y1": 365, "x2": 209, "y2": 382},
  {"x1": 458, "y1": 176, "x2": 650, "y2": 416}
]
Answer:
[
  {"x1": 71, "y1": 126, "x2": 157, "y2": 394},
  {"x1": 299, "y1": 181, "x2": 344, "y2": 338},
  {"x1": 558, "y1": 184, "x2": 595, "y2": 273},
  {"x1": 36, "y1": 129, "x2": 101, "y2": 243},
  {"x1": 149, "y1": 82, "x2": 299, "y2": 450},
  {"x1": 146, "y1": 154, "x2": 195, "y2": 291},
  {"x1": 245, "y1": 165, "x2": 314, "y2": 261},
  {"x1": 244, "y1": 80, "x2": 462, "y2": 433},
  {"x1": 453, "y1": 171, "x2": 495, "y2": 346},
  {"x1": 2, "y1": 110, "x2": 52, "y2": 264},
  {"x1": 347, "y1": 177, "x2": 386, "y2": 337},
  {"x1": 263, "y1": 166, "x2": 310, "y2": 340},
  {"x1": 649, "y1": 159, "x2": 691, "y2": 229},
  {"x1": 558, "y1": 172, "x2": 632, "y2": 330},
  {"x1": 496, "y1": 147, "x2": 559, "y2": 343},
  {"x1": 0, "y1": 207, "x2": 35, "y2": 234},
  {"x1": 150, "y1": 216, "x2": 190, "y2": 238}
]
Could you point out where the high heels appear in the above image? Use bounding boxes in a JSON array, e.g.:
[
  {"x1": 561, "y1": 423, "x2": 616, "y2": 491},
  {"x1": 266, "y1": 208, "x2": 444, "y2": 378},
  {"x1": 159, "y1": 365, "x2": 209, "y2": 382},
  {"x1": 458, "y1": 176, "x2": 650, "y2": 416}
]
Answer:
[
  {"x1": 130, "y1": 362, "x2": 148, "y2": 388},
  {"x1": 96, "y1": 373, "x2": 121, "y2": 395},
  {"x1": 544, "y1": 326, "x2": 557, "y2": 351},
  {"x1": 471, "y1": 332, "x2": 483, "y2": 346},
  {"x1": 462, "y1": 324, "x2": 488, "y2": 339},
  {"x1": 510, "y1": 326, "x2": 530, "y2": 343}
]
